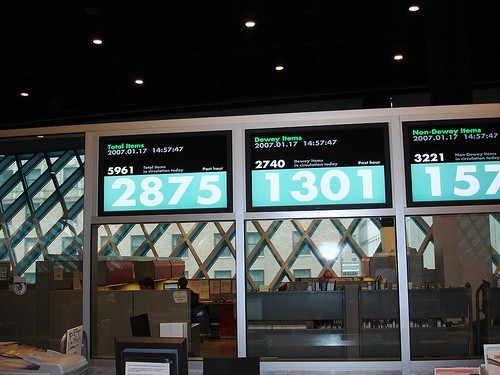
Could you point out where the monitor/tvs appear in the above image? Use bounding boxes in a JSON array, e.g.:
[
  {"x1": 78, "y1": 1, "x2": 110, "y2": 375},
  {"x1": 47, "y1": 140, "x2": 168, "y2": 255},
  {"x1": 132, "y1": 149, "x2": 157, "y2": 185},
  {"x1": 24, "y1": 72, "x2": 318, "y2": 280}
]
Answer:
[{"x1": 163, "y1": 282, "x2": 177, "y2": 289}]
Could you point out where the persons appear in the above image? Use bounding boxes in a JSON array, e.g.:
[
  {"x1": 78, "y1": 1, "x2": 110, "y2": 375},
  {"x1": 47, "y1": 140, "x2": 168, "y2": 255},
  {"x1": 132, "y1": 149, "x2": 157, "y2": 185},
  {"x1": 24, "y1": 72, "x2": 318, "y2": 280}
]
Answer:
[
  {"x1": 324, "y1": 270, "x2": 333, "y2": 277},
  {"x1": 177, "y1": 277, "x2": 200, "y2": 323},
  {"x1": 139, "y1": 278, "x2": 154, "y2": 290}
]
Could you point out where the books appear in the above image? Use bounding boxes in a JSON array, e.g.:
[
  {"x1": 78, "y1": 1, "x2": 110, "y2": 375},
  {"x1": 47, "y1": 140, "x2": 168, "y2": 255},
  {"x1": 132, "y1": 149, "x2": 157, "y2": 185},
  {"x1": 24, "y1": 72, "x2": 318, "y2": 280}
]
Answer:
[{"x1": 434, "y1": 350, "x2": 500, "y2": 375}]
